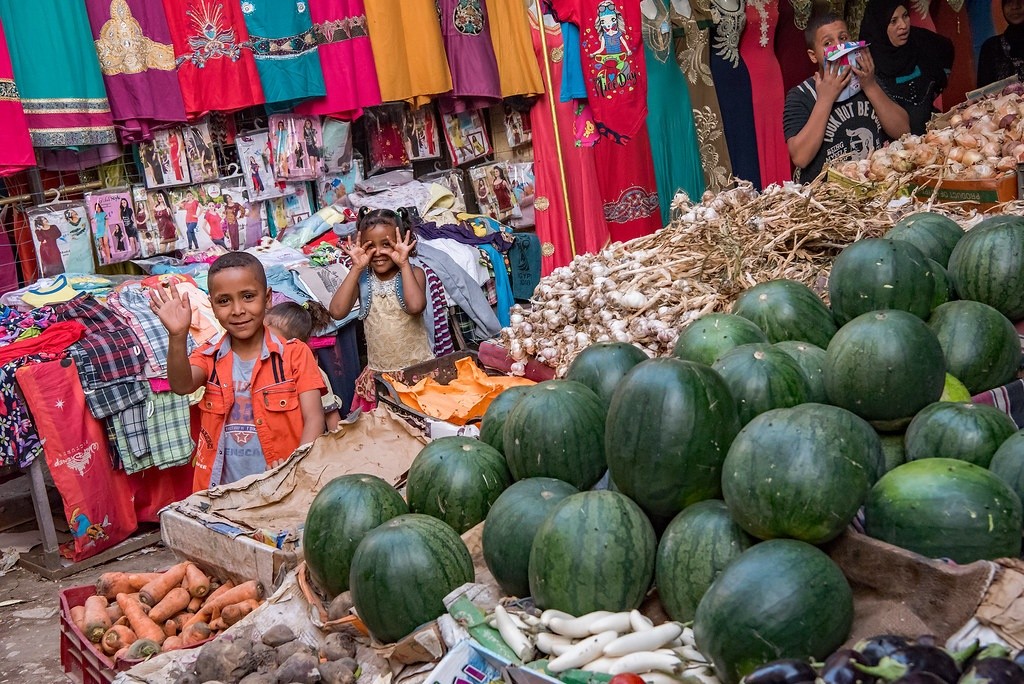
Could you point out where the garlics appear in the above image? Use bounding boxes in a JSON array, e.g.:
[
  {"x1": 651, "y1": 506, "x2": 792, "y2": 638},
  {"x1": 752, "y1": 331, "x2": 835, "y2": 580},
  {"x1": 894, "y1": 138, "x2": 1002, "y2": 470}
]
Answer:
[{"x1": 498, "y1": 179, "x2": 805, "y2": 375}]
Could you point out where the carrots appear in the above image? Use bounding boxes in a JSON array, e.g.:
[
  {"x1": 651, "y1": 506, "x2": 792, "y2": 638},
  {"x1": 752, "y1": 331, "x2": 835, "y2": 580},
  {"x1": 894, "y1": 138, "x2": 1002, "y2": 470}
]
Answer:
[{"x1": 69, "y1": 557, "x2": 259, "y2": 668}]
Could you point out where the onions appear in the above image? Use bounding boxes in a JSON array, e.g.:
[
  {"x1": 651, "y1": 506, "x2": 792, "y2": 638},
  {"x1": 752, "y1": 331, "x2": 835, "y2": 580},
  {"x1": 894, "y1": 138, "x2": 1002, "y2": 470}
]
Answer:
[{"x1": 837, "y1": 82, "x2": 1024, "y2": 178}]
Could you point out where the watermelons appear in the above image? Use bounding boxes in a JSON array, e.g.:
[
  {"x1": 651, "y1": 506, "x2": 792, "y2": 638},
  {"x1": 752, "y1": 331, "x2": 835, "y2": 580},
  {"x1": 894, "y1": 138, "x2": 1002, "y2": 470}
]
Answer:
[{"x1": 302, "y1": 214, "x2": 1023, "y2": 684}]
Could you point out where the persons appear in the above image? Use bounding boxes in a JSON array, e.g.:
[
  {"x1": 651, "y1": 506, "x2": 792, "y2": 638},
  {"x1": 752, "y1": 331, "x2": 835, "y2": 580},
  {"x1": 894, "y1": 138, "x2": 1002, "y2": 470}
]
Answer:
[
  {"x1": 976, "y1": 0, "x2": 1024, "y2": 90},
  {"x1": 859, "y1": 1, "x2": 954, "y2": 147},
  {"x1": 261, "y1": 301, "x2": 345, "y2": 435},
  {"x1": 149, "y1": 252, "x2": 328, "y2": 494},
  {"x1": 780, "y1": 10, "x2": 915, "y2": 182},
  {"x1": 327, "y1": 205, "x2": 436, "y2": 411},
  {"x1": 30, "y1": 106, "x2": 537, "y2": 269}
]
